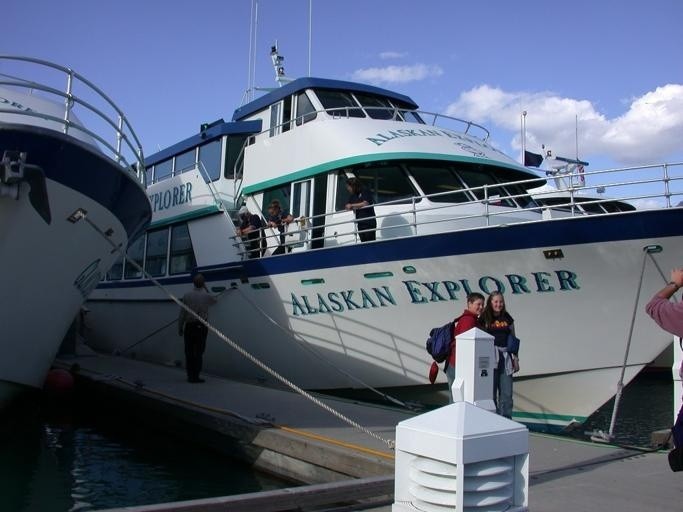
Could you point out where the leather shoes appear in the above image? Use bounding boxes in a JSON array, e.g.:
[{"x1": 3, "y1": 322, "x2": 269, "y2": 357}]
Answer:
[{"x1": 187, "y1": 377, "x2": 205, "y2": 384}]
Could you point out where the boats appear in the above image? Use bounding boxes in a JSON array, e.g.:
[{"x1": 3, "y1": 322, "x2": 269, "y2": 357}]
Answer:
[
  {"x1": 0, "y1": 55, "x2": 154, "y2": 396},
  {"x1": 79, "y1": 0, "x2": 682, "y2": 442}
]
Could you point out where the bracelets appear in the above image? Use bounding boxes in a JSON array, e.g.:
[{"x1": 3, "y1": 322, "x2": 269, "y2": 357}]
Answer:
[{"x1": 668, "y1": 280, "x2": 680, "y2": 291}]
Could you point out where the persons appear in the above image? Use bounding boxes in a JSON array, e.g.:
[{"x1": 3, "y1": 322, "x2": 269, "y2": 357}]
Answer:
[
  {"x1": 344, "y1": 178, "x2": 377, "y2": 242},
  {"x1": 447, "y1": 291, "x2": 519, "y2": 419},
  {"x1": 645, "y1": 268, "x2": 683, "y2": 472},
  {"x1": 178, "y1": 274, "x2": 217, "y2": 383},
  {"x1": 235, "y1": 206, "x2": 267, "y2": 257},
  {"x1": 268, "y1": 200, "x2": 294, "y2": 256}
]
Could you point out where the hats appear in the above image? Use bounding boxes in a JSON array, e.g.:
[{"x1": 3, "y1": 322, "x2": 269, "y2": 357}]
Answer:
[{"x1": 238, "y1": 206, "x2": 249, "y2": 215}]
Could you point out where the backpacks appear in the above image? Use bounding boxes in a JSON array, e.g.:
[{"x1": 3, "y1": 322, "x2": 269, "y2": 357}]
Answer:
[{"x1": 425, "y1": 314, "x2": 464, "y2": 363}]
